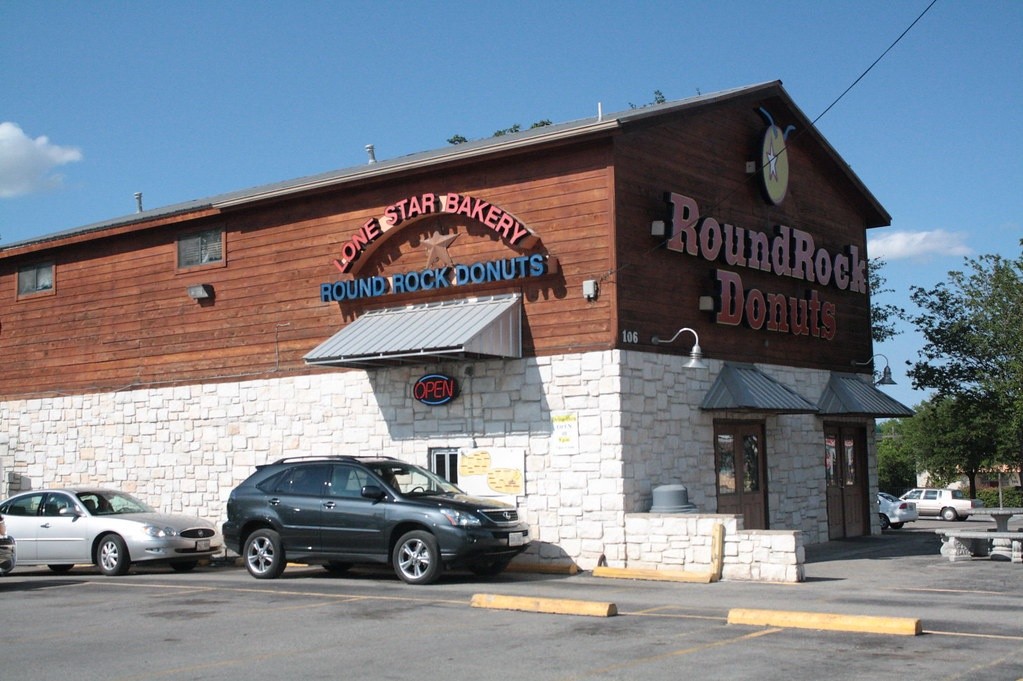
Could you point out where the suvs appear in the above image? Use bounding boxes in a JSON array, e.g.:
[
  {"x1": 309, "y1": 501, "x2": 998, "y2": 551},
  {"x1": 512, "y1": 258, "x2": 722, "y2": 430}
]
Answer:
[{"x1": 222, "y1": 455, "x2": 532, "y2": 586}]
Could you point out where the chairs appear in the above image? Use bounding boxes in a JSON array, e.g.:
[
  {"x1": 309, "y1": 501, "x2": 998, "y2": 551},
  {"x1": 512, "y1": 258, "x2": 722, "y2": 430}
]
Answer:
[
  {"x1": 83, "y1": 499, "x2": 97, "y2": 514},
  {"x1": 333, "y1": 472, "x2": 354, "y2": 498},
  {"x1": 1, "y1": 505, "x2": 26, "y2": 515},
  {"x1": 367, "y1": 474, "x2": 383, "y2": 495},
  {"x1": 45, "y1": 503, "x2": 60, "y2": 516}
]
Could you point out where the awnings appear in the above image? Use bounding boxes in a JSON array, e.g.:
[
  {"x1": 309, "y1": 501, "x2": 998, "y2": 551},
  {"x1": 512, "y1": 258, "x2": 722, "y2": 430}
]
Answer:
[
  {"x1": 818, "y1": 373, "x2": 917, "y2": 418},
  {"x1": 303, "y1": 293, "x2": 521, "y2": 368},
  {"x1": 699, "y1": 364, "x2": 822, "y2": 414}
]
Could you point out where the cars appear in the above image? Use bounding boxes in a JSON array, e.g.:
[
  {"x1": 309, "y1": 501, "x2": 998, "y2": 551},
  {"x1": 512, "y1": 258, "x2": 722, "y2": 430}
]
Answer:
[
  {"x1": 1, "y1": 486, "x2": 224, "y2": 577},
  {"x1": 877, "y1": 492, "x2": 919, "y2": 531}
]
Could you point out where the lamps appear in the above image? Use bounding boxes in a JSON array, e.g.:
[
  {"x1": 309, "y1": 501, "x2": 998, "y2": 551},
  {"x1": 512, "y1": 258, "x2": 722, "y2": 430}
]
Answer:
[
  {"x1": 652, "y1": 328, "x2": 708, "y2": 370},
  {"x1": 185, "y1": 284, "x2": 214, "y2": 300},
  {"x1": 852, "y1": 354, "x2": 897, "y2": 385}
]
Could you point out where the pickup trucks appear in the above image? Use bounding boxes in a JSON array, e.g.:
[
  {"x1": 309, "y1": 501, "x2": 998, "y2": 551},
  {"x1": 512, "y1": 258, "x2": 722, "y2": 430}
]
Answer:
[{"x1": 897, "y1": 487, "x2": 984, "y2": 522}]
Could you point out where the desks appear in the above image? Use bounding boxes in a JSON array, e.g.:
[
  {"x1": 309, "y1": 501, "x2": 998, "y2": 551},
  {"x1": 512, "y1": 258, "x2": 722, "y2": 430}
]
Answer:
[{"x1": 967, "y1": 508, "x2": 1023, "y2": 531}]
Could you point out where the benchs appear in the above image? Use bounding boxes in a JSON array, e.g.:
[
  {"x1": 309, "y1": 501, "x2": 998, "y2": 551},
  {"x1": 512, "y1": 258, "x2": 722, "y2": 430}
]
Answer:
[
  {"x1": 945, "y1": 531, "x2": 1023, "y2": 563},
  {"x1": 935, "y1": 527, "x2": 997, "y2": 557}
]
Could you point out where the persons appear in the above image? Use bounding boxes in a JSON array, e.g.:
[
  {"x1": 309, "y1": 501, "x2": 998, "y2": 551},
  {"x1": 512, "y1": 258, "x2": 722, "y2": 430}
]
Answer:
[
  {"x1": 380, "y1": 471, "x2": 401, "y2": 495},
  {"x1": 96, "y1": 496, "x2": 113, "y2": 513}
]
